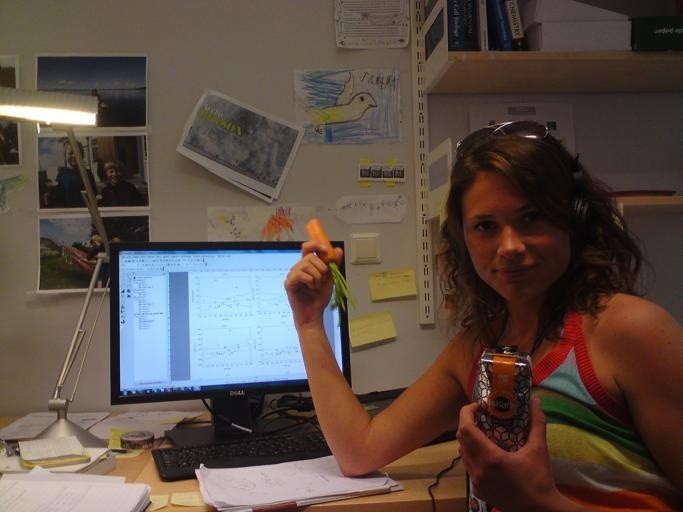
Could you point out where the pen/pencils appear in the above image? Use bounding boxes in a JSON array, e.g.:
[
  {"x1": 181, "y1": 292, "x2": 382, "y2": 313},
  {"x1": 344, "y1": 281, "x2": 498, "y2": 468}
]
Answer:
[{"x1": 110, "y1": 449, "x2": 133, "y2": 453}]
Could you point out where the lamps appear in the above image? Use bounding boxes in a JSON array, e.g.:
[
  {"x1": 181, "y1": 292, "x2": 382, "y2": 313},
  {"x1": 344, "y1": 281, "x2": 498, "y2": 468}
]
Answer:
[{"x1": 0, "y1": 86, "x2": 114, "y2": 450}]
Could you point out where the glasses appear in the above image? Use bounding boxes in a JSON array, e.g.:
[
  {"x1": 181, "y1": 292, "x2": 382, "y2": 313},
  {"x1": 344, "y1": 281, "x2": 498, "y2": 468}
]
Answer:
[{"x1": 457, "y1": 120, "x2": 549, "y2": 149}]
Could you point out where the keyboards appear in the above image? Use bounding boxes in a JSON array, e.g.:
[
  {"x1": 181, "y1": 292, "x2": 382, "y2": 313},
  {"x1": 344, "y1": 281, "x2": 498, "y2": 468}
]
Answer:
[{"x1": 150, "y1": 431, "x2": 333, "y2": 482}]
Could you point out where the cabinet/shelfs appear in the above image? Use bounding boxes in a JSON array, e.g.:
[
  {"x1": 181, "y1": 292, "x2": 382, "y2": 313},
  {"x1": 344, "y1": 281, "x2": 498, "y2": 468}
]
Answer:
[{"x1": 411, "y1": 0, "x2": 683, "y2": 327}]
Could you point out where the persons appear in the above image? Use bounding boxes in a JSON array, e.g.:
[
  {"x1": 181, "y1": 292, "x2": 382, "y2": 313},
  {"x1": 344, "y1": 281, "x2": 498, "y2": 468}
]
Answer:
[
  {"x1": 101, "y1": 161, "x2": 145, "y2": 207},
  {"x1": 60, "y1": 234, "x2": 123, "y2": 289},
  {"x1": 54, "y1": 140, "x2": 97, "y2": 208},
  {"x1": 284, "y1": 120, "x2": 683, "y2": 512}
]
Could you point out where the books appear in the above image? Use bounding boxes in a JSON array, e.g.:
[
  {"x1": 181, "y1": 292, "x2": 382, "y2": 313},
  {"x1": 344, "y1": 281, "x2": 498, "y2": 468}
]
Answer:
[
  {"x1": 195, "y1": 454, "x2": 405, "y2": 512},
  {"x1": 446, "y1": 0, "x2": 529, "y2": 51},
  {"x1": 1, "y1": 436, "x2": 152, "y2": 512}
]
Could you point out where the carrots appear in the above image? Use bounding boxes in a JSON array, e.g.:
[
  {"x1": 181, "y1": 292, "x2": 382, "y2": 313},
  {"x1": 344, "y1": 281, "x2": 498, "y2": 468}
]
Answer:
[{"x1": 305, "y1": 219, "x2": 341, "y2": 267}]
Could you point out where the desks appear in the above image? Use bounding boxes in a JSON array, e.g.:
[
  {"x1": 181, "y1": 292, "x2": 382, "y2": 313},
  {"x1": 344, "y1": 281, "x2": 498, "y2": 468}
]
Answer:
[{"x1": 0, "y1": 413, "x2": 468, "y2": 512}]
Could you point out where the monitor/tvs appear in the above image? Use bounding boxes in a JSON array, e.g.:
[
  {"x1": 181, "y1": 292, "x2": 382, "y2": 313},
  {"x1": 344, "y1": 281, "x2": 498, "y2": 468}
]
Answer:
[{"x1": 107, "y1": 240, "x2": 351, "y2": 448}]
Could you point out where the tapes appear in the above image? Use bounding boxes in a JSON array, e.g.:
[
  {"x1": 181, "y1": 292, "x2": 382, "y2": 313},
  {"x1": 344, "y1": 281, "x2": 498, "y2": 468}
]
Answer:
[{"x1": 120, "y1": 431, "x2": 153, "y2": 449}]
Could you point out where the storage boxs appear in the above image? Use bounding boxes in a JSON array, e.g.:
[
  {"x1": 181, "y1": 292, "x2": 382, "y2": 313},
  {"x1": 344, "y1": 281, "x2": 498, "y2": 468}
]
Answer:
[
  {"x1": 528, "y1": 21, "x2": 631, "y2": 50},
  {"x1": 523, "y1": 0, "x2": 628, "y2": 32},
  {"x1": 630, "y1": 15, "x2": 683, "y2": 50}
]
